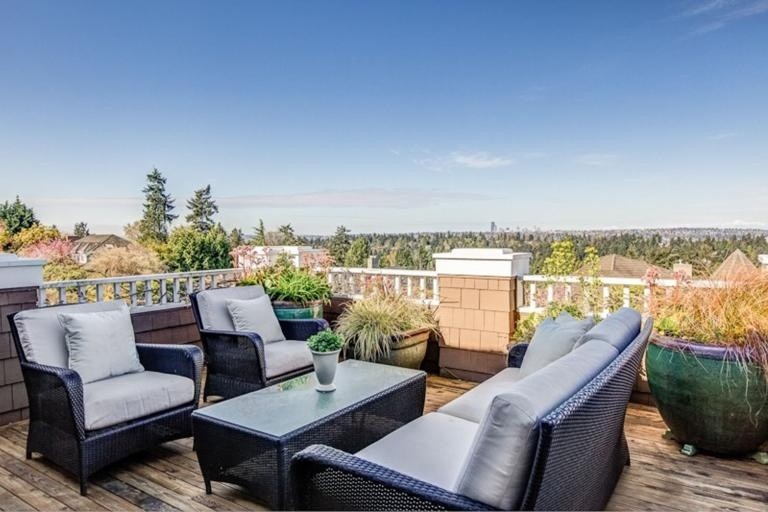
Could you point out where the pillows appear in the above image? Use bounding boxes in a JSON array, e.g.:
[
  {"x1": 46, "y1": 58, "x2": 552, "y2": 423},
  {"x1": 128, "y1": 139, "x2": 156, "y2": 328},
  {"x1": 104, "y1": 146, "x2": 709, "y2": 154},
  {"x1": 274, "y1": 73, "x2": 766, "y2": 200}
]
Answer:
[
  {"x1": 517, "y1": 317, "x2": 585, "y2": 380},
  {"x1": 57, "y1": 308, "x2": 145, "y2": 385},
  {"x1": 225, "y1": 294, "x2": 285, "y2": 344},
  {"x1": 555, "y1": 313, "x2": 594, "y2": 331}
]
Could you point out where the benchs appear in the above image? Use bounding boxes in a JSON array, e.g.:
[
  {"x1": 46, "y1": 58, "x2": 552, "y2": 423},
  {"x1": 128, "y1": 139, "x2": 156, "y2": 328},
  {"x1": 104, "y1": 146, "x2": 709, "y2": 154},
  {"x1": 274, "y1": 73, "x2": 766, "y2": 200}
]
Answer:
[{"x1": 288, "y1": 310, "x2": 654, "y2": 512}]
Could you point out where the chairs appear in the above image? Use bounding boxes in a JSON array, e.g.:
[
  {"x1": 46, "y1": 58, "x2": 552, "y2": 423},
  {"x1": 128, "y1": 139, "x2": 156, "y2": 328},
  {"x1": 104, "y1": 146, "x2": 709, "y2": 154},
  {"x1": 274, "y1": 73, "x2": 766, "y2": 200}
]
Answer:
[
  {"x1": 7, "y1": 300, "x2": 204, "y2": 496},
  {"x1": 188, "y1": 284, "x2": 332, "y2": 403}
]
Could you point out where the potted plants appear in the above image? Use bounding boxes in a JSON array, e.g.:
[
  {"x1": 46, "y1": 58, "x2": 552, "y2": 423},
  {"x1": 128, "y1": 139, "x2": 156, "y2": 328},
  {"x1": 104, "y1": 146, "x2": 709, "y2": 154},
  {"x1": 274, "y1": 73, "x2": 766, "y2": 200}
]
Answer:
[
  {"x1": 306, "y1": 331, "x2": 344, "y2": 393},
  {"x1": 329, "y1": 284, "x2": 442, "y2": 370},
  {"x1": 644, "y1": 263, "x2": 768, "y2": 466},
  {"x1": 236, "y1": 256, "x2": 334, "y2": 319}
]
